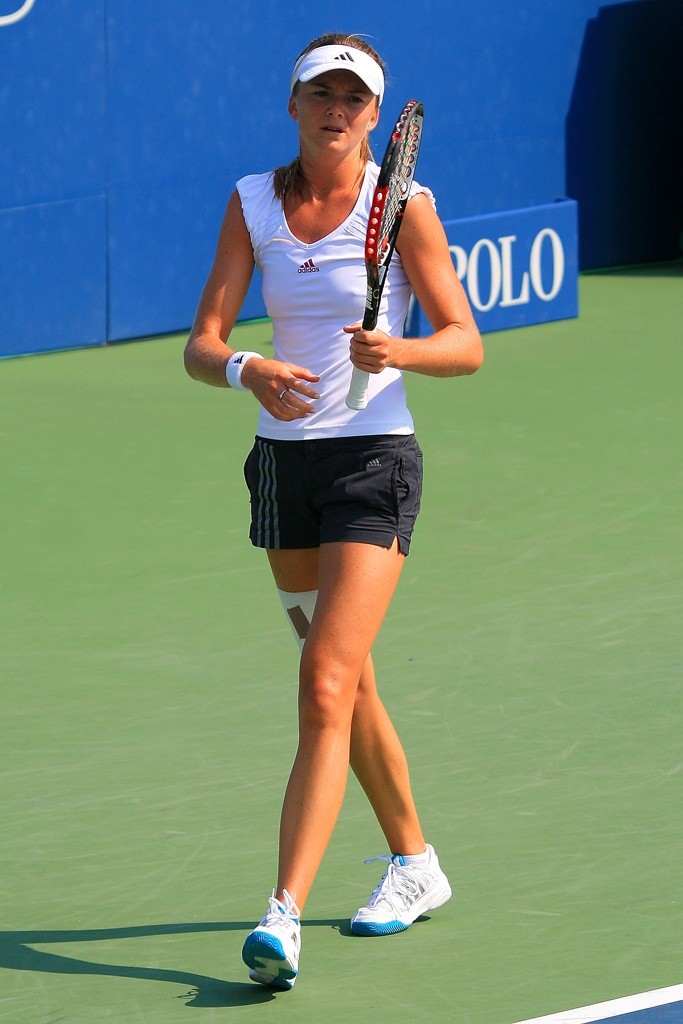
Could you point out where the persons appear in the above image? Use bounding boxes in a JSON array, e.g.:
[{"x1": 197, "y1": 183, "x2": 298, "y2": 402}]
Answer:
[{"x1": 184, "y1": 32, "x2": 485, "y2": 992}]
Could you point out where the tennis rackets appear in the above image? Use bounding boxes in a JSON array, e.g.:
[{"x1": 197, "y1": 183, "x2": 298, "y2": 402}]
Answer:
[{"x1": 343, "y1": 98, "x2": 427, "y2": 414}]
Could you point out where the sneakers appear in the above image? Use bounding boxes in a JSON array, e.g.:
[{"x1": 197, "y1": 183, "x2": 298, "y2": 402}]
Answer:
[
  {"x1": 350, "y1": 843, "x2": 452, "y2": 934},
  {"x1": 241, "y1": 886, "x2": 303, "y2": 991}
]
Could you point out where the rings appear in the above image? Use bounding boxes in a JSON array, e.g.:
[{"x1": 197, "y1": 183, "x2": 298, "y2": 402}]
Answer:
[{"x1": 279, "y1": 389, "x2": 287, "y2": 400}]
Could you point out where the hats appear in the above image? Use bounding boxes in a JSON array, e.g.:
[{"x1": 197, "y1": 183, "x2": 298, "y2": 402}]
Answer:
[{"x1": 290, "y1": 44, "x2": 385, "y2": 106}]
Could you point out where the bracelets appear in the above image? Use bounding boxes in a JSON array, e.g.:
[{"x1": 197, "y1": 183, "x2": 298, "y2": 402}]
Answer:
[{"x1": 225, "y1": 351, "x2": 265, "y2": 390}]
